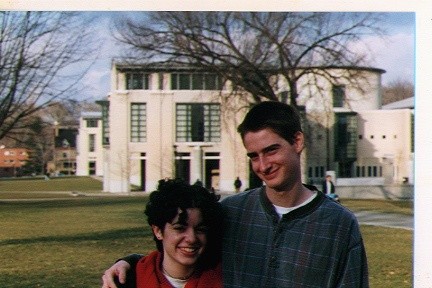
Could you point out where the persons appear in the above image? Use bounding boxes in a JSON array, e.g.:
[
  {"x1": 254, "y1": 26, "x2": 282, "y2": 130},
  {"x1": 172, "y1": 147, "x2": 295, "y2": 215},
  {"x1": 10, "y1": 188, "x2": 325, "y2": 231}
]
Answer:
[
  {"x1": 107, "y1": 177, "x2": 225, "y2": 288},
  {"x1": 321, "y1": 174, "x2": 335, "y2": 195},
  {"x1": 233, "y1": 176, "x2": 242, "y2": 194},
  {"x1": 100, "y1": 99, "x2": 372, "y2": 288},
  {"x1": 401, "y1": 176, "x2": 409, "y2": 186}
]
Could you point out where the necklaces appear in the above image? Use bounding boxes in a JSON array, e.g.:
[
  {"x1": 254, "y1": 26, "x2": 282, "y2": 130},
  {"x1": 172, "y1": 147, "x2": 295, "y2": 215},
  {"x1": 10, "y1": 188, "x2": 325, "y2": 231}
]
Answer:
[{"x1": 161, "y1": 264, "x2": 194, "y2": 280}]
